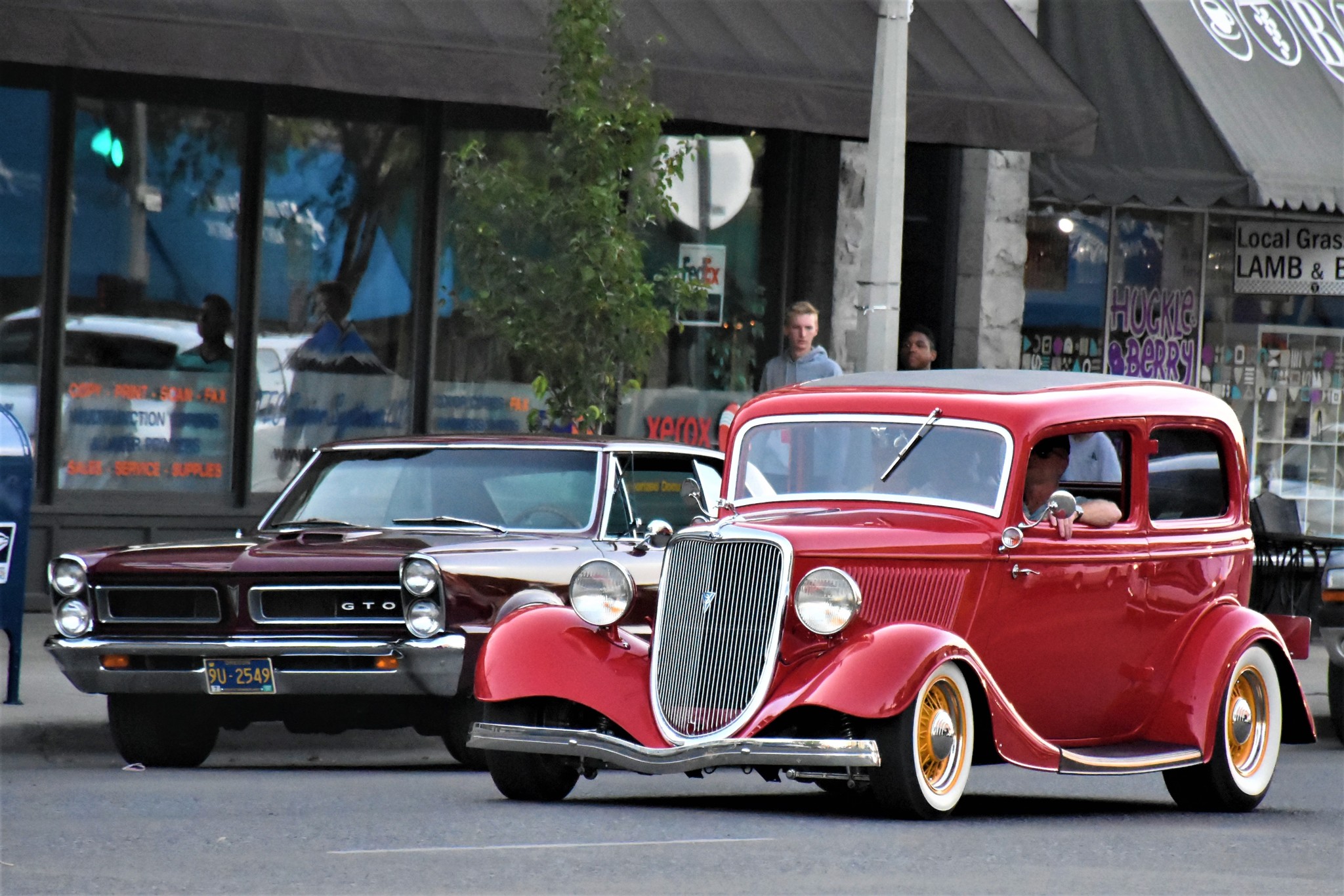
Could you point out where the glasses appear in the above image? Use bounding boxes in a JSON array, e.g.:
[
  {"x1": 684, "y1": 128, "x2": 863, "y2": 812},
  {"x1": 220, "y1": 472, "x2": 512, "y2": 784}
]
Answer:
[{"x1": 1030, "y1": 446, "x2": 1068, "y2": 462}]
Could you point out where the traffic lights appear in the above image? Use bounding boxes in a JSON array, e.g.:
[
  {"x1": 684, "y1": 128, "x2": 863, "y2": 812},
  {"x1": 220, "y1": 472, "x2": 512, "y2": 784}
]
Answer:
[{"x1": 92, "y1": 118, "x2": 135, "y2": 182}]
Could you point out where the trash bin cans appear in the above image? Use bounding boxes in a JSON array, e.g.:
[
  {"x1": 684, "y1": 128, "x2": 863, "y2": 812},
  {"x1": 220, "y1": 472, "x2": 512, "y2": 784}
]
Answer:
[{"x1": 0, "y1": 404, "x2": 32, "y2": 631}]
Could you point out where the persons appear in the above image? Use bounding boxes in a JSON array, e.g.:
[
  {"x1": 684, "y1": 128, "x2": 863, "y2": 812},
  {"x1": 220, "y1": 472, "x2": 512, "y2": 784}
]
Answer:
[
  {"x1": 759, "y1": 301, "x2": 849, "y2": 492},
  {"x1": 167, "y1": 295, "x2": 261, "y2": 458},
  {"x1": 277, "y1": 282, "x2": 391, "y2": 485},
  {"x1": 907, "y1": 435, "x2": 1122, "y2": 541},
  {"x1": 1059, "y1": 431, "x2": 1121, "y2": 483},
  {"x1": 875, "y1": 325, "x2": 938, "y2": 493}
]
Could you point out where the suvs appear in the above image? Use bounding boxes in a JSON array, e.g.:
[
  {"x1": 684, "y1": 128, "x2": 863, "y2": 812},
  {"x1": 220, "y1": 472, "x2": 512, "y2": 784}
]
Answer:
[{"x1": 0, "y1": 309, "x2": 313, "y2": 494}]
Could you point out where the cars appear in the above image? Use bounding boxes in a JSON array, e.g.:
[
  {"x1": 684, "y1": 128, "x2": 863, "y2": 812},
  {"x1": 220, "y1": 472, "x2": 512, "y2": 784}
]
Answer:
[
  {"x1": 42, "y1": 434, "x2": 776, "y2": 768},
  {"x1": 1321, "y1": 551, "x2": 1344, "y2": 744},
  {"x1": 1249, "y1": 423, "x2": 1344, "y2": 537}
]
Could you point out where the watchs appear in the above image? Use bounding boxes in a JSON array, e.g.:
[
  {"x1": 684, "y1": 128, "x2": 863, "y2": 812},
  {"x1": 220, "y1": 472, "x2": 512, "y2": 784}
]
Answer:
[{"x1": 1075, "y1": 505, "x2": 1083, "y2": 519}]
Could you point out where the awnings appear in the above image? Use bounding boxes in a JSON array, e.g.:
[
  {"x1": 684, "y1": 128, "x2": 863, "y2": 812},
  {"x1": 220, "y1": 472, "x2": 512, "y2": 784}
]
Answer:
[
  {"x1": 0, "y1": 0, "x2": 1101, "y2": 163},
  {"x1": 1029, "y1": 2, "x2": 1344, "y2": 212}
]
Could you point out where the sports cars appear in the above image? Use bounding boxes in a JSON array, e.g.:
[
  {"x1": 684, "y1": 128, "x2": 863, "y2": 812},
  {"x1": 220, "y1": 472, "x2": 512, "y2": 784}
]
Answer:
[{"x1": 467, "y1": 370, "x2": 1317, "y2": 825}]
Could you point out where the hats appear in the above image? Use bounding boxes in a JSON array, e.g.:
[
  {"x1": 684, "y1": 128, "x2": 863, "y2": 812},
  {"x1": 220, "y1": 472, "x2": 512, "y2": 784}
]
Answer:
[{"x1": 1033, "y1": 432, "x2": 1071, "y2": 457}]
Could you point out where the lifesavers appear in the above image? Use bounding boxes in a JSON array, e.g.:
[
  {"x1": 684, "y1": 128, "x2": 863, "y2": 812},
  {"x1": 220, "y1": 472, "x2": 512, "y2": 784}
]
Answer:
[{"x1": 719, "y1": 402, "x2": 752, "y2": 453}]
